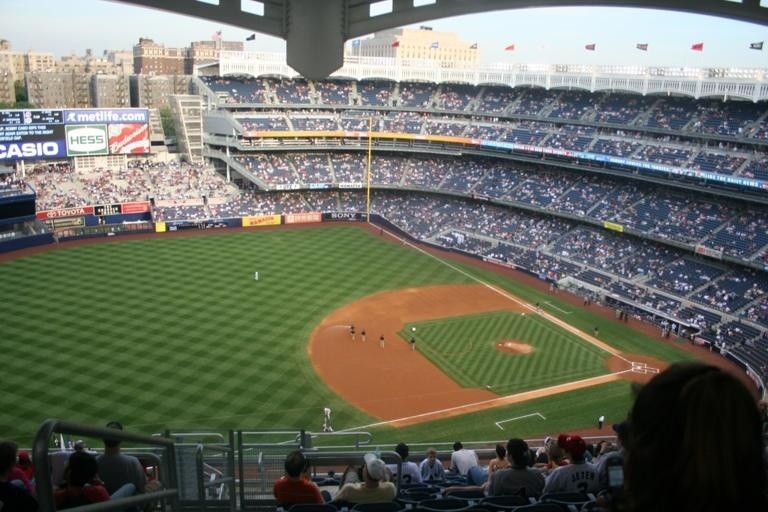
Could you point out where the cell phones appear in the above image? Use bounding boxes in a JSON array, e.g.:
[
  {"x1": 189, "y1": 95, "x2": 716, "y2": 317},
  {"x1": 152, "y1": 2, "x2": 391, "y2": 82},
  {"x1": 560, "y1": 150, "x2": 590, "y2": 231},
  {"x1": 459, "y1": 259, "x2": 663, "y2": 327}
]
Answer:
[{"x1": 604, "y1": 453, "x2": 624, "y2": 499}]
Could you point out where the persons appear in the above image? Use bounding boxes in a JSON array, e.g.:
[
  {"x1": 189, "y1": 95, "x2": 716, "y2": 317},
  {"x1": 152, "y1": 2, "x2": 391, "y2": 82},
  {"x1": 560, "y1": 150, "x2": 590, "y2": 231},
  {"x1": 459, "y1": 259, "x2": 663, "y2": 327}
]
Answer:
[
  {"x1": 96, "y1": 422, "x2": 148, "y2": 493},
  {"x1": 333, "y1": 453, "x2": 397, "y2": 507},
  {"x1": 201, "y1": 76, "x2": 372, "y2": 216},
  {"x1": 617, "y1": 363, "x2": 768, "y2": 510},
  {"x1": 442, "y1": 434, "x2": 599, "y2": 497},
  {"x1": 1, "y1": 156, "x2": 239, "y2": 234},
  {"x1": 391, "y1": 443, "x2": 422, "y2": 484},
  {"x1": 350, "y1": 331, "x2": 355, "y2": 339},
  {"x1": 598, "y1": 416, "x2": 605, "y2": 429},
  {"x1": 349, "y1": 324, "x2": 356, "y2": 330},
  {"x1": 359, "y1": 328, "x2": 366, "y2": 343},
  {"x1": 272, "y1": 451, "x2": 325, "y2": 506},
  {"x1": 379, "y1": 333, "x2": 385, "y2": 351},
  {"x1": 420, "y1": 448, "x2": 445, "y2": 482},
  {"x1": 402, "y1": 82, "x2": 768, "y2": 357},
  {"x1": 599, "y1": 423, "x2": 628, "y2": 489},
  {"x1": 1, "y1": 440, "x2": 162, "y2": 512}
]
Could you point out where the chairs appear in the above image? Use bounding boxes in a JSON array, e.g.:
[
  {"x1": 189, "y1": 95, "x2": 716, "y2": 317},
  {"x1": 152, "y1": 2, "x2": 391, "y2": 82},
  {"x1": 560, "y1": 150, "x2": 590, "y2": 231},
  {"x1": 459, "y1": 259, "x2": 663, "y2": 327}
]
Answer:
[{"x1": 276, "y1": 451, "x2": 624, "y2": 512}]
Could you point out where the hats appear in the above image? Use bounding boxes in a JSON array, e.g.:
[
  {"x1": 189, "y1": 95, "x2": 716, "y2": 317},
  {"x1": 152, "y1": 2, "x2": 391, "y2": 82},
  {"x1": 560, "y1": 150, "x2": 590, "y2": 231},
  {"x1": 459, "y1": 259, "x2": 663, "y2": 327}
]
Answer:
[
  {"x1": 508, "y1": 439, "x2": 529, "y2": 465},
  {"x1": 558, "y1": 434, "x2": 586, "y2": 453},
  {"x1": 364, "y1": 453, "x2": 386, "y2": 481}
]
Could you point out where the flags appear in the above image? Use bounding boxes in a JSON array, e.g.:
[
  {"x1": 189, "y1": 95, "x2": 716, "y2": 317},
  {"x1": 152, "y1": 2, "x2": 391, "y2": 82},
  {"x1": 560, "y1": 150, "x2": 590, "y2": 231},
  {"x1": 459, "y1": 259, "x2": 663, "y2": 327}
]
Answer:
[
  {"x1": 350, "y1": 38, "x2": 362, "y2": 49},
  {"x1": 689, "y1": 42, "x2": 705, "y2": 52},
  {"x1": 468, "y1": 42, "x2": 478, "y2": 52},
  {"x1": 391, "y1": 42, "x2": 399, "y2": 50},
  {"x1": 214, "y1": 30, "x2": 223, "y2": 42},
  {"x1": 748, "y1": 41, "x2": 764, "y2": 52},
  {"x1": 432, "y1": 42, "x2": 439, "y2": 50},
  {"x1": 635, "y1": 42, "x2": 648, "y2": 51},
  {"x1": 504, "y1": 42, "x2": 516, "y2": 52},
  {"x1": 582, "y1": 43, "x2": 598, "y2": 52},
  {"x1": 245, "y1": 32, "x2": 256, "y2": 44}
]
